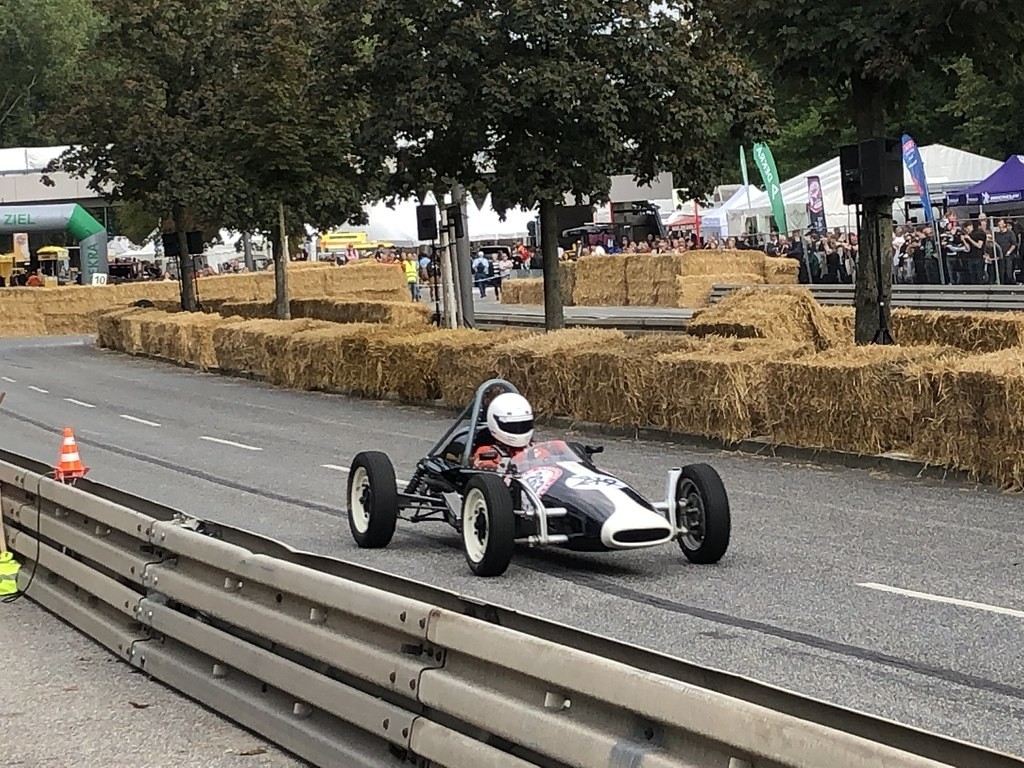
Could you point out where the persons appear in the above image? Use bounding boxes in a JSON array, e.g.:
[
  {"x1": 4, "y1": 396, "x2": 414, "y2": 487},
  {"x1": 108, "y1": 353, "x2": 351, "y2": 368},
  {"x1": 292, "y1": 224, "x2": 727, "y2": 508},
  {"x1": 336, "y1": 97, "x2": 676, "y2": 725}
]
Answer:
[
  {"x1": 474, "y1": 392, "x2": 552, "y2": 475},
  {"x1": 10, "y1": 243, "x2": 442, "y2": 303},
  {"x1": 470, "y1": 211, "x2": 1024, "y2": 300}
]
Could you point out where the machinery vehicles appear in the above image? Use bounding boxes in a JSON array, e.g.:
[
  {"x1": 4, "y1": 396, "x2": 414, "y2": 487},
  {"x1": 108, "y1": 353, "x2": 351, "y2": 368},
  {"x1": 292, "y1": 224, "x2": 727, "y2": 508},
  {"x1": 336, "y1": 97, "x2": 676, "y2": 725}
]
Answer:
[{"x1": 0, "y1": 245, "x2": 163, "y2": 288}]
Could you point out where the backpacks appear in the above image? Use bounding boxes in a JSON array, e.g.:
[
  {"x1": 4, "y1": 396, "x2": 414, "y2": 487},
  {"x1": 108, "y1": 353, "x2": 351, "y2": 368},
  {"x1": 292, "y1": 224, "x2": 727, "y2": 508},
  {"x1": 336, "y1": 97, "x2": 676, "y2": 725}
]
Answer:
[{"x1": 477, "y1": 259, "x2": 485, "y2": 273}]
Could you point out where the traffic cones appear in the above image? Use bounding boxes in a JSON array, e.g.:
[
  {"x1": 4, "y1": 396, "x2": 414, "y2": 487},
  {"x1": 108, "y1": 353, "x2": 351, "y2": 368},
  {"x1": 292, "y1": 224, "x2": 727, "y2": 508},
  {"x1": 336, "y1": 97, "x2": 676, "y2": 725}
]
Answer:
[{"x1": 53, "y1": 429, "x2": 85, "y2": 473}]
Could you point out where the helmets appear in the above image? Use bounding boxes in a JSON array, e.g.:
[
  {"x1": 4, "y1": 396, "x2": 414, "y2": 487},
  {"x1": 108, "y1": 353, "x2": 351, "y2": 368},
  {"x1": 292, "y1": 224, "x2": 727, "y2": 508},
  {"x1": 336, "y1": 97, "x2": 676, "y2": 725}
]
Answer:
[{"x1": 487, "y1": 392, "x2": 535, "y2": 447}]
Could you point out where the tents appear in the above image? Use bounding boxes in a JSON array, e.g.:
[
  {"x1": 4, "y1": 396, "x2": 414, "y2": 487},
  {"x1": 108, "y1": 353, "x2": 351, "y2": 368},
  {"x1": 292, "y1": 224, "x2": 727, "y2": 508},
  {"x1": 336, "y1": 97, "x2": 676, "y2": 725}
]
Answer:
[
  {"x1": 107, "y1": 212, "x2": 325, "y2": 274},
  {"x1": 660, "y1": 143, "x2": 1024, "y2": 245}
]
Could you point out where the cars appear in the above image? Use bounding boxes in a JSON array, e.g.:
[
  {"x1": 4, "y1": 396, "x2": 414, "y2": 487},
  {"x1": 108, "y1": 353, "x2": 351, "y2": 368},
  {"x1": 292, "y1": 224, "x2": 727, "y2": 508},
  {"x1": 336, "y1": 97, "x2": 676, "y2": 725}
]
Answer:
[{"x1": 345, "y1": 377, "x2": 731, "y2": 577}]
